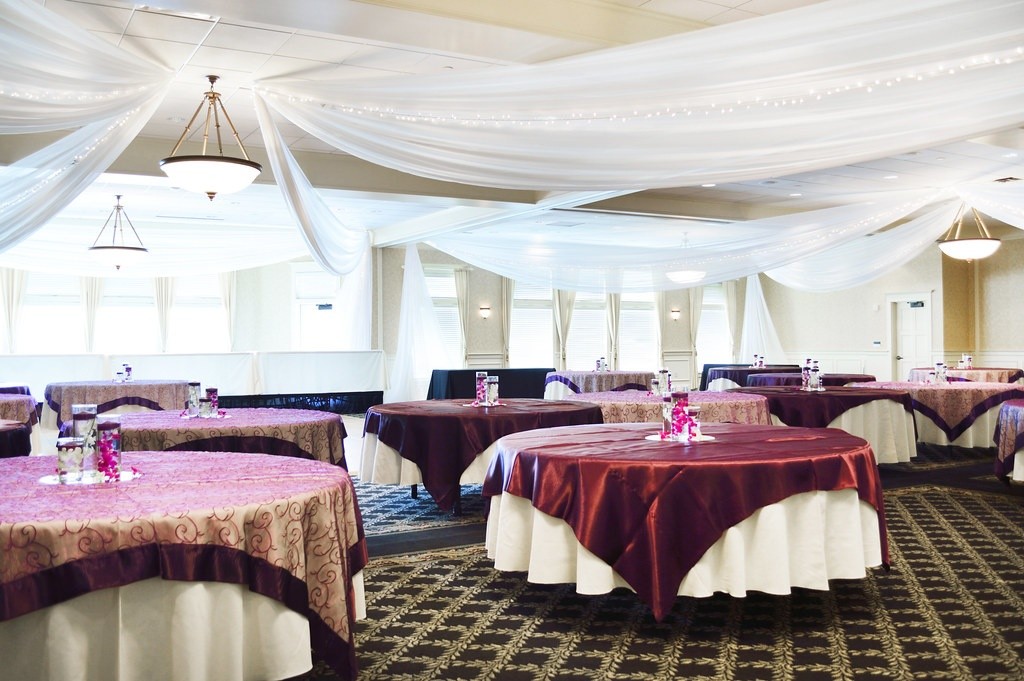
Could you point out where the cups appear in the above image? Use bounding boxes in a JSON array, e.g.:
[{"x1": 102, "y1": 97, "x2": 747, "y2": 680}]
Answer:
[
  {"x1": 595, "y1": 357, "x2": 608, "y2": 372},
  {"x1": 753, "y1": 354, "x2": 764, "y2": 369},
  {"x1": 476, "y1": 371, "x2": 499, "y2": 406},
  {"x1": 801, "y1": 358, "x2": 822, "y2": 391},
  {"x1": 958, "y1": 353, "x2": 973, "y2": 370},
  {"x1": 187, "y1": 382, "x2": 219, "y2": 420},
  {"x1": 56, "y1": 403, "x2": 121, "y2": 485},
  {"x1": 650, "y1": 369, "x2": 673, "y2": 397},
  {"x1": 928, "y1": 363, "x2": 948, "y2": 386},
  {"x1": 661, "y1": 391, "x2": 701, "y2": 443}
]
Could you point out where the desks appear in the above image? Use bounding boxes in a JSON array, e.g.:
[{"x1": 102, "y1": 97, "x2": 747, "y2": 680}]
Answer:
[{"x1": 0, "y1": 366, "x2": 1024, "y2": 681}]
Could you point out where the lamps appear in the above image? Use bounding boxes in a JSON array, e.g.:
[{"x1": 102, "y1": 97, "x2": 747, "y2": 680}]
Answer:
[
  {"x1": 480, "y1": 308, "x2": 491, "y2": 318},
  {"x1": 937, "y1": 202, "x2": 1003, "y2": 265},
  {"x1": 87, "y1": 194, "x2": 148, "y2": 270},
  {"x1": 672, "y1": 310, "x2": 680, "y2": 321},
  {"x1": 158, "y1": 74, "x2": 263, "y2": 201}
]
